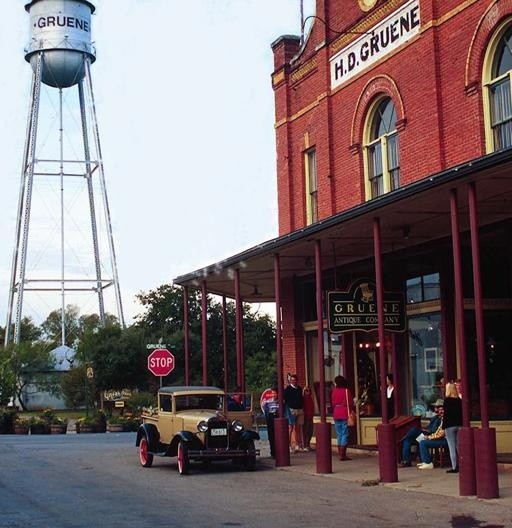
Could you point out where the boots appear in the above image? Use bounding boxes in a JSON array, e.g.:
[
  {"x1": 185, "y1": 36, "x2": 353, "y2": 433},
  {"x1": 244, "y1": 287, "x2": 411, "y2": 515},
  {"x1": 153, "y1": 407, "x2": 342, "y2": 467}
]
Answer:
[{"x1": 338, "y1": 446, "x2": 352, "y2": 460}]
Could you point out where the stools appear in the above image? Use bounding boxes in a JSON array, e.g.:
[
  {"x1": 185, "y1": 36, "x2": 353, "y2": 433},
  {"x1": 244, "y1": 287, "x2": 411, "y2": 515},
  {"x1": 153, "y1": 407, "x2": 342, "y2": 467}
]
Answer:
[{"x1": 431, "y1": 445, "x2": 451, "y2": 468}]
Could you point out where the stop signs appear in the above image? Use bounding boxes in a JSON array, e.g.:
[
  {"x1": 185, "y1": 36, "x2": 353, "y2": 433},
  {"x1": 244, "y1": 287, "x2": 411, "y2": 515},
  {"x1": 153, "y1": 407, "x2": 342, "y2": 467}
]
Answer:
[
  {"x1": 148, "y1": 348, "x2": 175, "y2": 375},
  {"x1": 261, "y1": 388, "x2": 273, "y2": 400}
]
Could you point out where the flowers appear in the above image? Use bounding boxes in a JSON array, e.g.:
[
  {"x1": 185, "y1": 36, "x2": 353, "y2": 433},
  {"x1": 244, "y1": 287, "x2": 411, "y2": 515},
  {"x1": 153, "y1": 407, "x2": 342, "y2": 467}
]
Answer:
[{"x1": 14, "y1": 407, "x2": 127, "y2": 427}]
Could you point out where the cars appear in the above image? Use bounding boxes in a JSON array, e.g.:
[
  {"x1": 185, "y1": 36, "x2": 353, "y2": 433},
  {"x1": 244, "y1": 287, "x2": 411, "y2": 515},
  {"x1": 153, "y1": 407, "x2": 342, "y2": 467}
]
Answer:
[{"x1": 135, "y1": 385, "x2": 261, "y2": 474}]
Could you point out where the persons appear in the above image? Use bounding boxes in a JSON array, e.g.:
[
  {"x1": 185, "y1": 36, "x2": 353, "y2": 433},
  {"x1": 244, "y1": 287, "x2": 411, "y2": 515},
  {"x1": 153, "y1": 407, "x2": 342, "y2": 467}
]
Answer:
[
  {"x1": 302, "y1": 385, "x2": 316, "y2": 453},
  {"x1": 227, "y1": 395, "x2": 240, "y2": 410},
  {"x1": 441, "y1": 382, "x2": 463, "y2": 473},
  {"x1": 330, "y1": 375, "x2": 354, "y2": 461},
  {"x1": 416, "y1": 405, "x2": 445, "y2": 469},
  {"x1": 262, "y1": 387, "x2": 278, "y2": 402},
  {"x1": 284, "y1": 374, "x2": 304, "y2": 454},
  {"x1": 386, "y1": 373, "x2": 395, "y2": 419},
  {"x1": 397, "y1": 399, "x2": 444, "y2": 467}
]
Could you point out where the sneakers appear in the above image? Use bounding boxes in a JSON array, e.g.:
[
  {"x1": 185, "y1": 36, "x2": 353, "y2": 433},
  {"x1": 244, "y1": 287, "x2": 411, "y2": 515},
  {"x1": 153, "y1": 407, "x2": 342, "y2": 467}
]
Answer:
[
  {"x1": 294, "y1": 445, "x2": 314, "y2": 453},
  {"x1": 416, "y1": 462, "x2": 434, "y2": 469},
  {"x1": 399, "y1": 462, "x2": 411, "y2": 467}
]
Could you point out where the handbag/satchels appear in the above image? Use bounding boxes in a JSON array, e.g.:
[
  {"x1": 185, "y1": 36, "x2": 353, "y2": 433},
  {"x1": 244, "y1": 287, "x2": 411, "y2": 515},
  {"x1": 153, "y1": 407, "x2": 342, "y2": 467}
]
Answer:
[{"x1": 348, "y1": 410, "x2": 356, "y2": 426}]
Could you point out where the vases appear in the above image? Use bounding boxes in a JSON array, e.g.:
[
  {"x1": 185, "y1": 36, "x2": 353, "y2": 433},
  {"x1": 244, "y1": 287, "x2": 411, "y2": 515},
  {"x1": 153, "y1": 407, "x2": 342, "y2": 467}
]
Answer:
[{"x1": 16, "y1": 424, "x2": 122, "y2": 434}]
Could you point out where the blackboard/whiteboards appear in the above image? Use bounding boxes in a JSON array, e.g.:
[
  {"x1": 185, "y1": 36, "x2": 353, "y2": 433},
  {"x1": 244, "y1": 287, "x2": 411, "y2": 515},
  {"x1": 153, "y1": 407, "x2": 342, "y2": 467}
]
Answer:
[{"x1": 326, "y1": 274, "x2": 407, "y2": 334}]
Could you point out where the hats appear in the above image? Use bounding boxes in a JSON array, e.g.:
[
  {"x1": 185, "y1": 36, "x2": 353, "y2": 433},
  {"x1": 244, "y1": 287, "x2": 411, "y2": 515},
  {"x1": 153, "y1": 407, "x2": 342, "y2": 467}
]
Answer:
[{"x1": 431, "y1": 399, "x2": 444, "y2": 406}]
